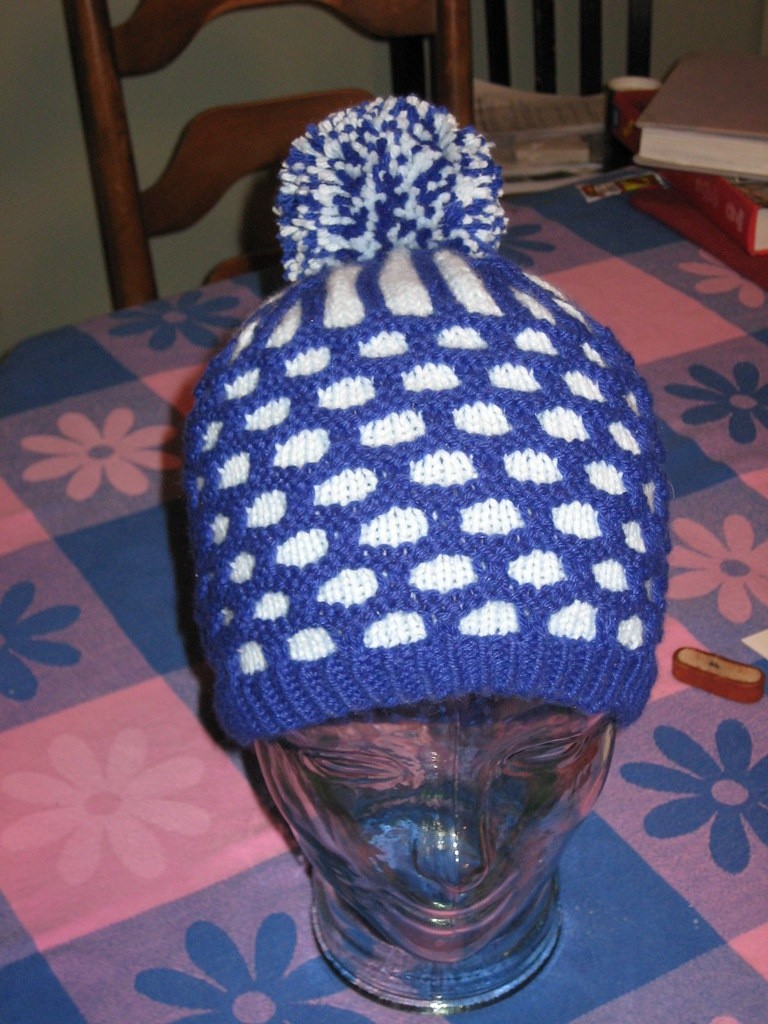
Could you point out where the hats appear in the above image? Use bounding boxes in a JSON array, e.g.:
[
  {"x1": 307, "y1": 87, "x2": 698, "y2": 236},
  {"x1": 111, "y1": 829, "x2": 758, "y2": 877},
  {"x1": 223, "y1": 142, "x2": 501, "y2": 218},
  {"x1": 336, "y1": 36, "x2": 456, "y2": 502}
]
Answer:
[{"x1": 183, "y1": 96, "x2": 672, "y2": 750}]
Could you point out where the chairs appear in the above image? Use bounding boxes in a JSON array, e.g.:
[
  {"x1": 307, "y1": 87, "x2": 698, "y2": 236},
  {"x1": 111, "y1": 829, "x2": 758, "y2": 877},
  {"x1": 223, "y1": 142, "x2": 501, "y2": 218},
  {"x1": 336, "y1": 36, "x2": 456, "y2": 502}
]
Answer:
[{"x1": 62, "y1": 1, "x2": 474, "y2": 313}]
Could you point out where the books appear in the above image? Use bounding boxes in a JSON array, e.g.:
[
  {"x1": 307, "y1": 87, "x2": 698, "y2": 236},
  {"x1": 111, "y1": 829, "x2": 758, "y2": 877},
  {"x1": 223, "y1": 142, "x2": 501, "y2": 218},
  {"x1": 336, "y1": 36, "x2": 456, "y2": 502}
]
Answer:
[{"x1": 473, "y1": 50, "x2": 768, "y2": 292}]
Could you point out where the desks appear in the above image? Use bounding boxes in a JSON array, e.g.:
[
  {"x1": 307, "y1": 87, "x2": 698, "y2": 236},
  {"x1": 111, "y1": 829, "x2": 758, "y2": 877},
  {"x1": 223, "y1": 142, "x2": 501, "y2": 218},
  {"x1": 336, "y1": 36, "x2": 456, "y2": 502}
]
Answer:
[{"x1": 0, "y1": 187, "x2": 768, "y2": 1023}]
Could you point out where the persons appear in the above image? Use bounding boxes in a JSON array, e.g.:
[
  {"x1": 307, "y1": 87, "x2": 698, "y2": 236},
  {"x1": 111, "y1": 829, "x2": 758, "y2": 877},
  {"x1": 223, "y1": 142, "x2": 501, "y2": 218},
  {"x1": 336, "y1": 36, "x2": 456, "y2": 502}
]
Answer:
[{"x1": 184, "y1": 96, "x2": 668, "y2": 1013}]
